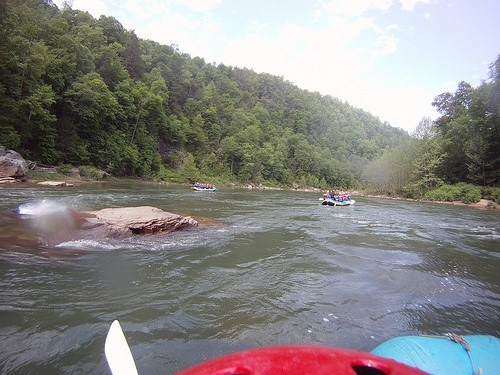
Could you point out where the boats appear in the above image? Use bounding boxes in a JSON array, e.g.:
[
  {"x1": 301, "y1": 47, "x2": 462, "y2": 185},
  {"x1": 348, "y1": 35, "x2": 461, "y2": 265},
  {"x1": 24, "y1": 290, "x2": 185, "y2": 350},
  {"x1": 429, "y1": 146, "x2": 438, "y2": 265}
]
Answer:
[
  {"x1": 175, "y1": 344, "x2": 428, "y2": 375},
  {"x1": 371, "y1": 334, "x2": 500, "y2": 375},
  {"x1": 190, "y1": 185, "x2": 218, "y2": 192},
  {"x1": 322, "y1": 197, "x2": 356, "y2": 206}
]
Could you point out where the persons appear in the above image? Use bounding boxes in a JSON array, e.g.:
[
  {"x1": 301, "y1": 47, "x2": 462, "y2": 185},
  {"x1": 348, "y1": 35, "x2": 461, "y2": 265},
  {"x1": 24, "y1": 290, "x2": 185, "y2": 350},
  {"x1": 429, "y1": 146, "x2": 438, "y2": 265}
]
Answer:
[
  {"x1": 330, "y1": 189, "x2": 336, "y2": 201},
  {"x1": 323, "y1": 194, "x2": 351, "y2": 202},
  {"x1": 199, "y1": 183, "x2": 213, "y2": 189}
]
